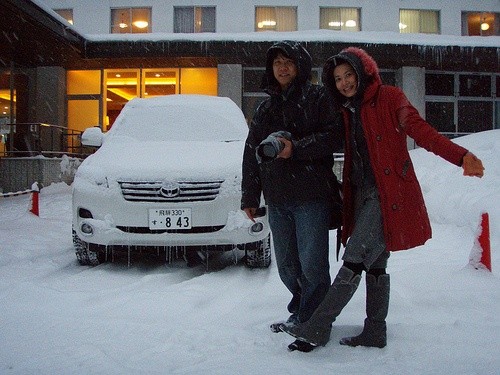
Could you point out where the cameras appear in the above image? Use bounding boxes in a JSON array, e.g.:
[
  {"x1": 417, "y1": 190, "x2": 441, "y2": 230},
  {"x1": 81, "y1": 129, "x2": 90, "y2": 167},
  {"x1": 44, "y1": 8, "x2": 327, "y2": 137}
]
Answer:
[{"x1": 256, "y1": 130, "x2": 292, "y2": 162}]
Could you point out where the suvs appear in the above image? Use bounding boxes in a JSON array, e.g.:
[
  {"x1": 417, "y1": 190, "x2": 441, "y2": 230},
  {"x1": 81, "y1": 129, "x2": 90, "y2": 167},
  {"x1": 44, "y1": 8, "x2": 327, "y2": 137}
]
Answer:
[{"x1": 70, "y1": 94, "x2": 272, "y2": 270}]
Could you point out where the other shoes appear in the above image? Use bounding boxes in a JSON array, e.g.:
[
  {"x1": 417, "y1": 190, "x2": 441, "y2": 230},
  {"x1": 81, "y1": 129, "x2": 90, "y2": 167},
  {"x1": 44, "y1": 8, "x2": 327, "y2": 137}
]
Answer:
[
  {"x1": 270, "y1": 321, "x2": 296, "y2": 333},
  {"x1": 288, "y1": 338, "x2": 317, "y2": 352}
]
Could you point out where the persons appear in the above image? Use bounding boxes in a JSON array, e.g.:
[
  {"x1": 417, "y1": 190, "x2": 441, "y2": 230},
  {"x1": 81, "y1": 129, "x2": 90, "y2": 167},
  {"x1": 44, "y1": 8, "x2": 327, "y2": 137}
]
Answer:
[
  {"x1": 240, "y1": 41, "x2": 344, "y2": 353},
  {"x1": 280, "y1": 48, "x2": 484, "y2": 349}
]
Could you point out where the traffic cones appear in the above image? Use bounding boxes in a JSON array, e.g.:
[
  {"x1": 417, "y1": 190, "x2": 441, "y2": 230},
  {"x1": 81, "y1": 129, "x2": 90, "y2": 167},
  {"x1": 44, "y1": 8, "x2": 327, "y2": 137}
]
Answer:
[{"x1": 466, "y1": 212, "x2": 491, "y2": 273}]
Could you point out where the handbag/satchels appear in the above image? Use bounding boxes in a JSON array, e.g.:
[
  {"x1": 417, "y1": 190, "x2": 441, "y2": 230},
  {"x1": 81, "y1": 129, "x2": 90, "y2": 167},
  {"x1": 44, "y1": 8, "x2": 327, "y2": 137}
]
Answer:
[{"x1": 324, "y1": 175, "x2": 343, "y2": 231}]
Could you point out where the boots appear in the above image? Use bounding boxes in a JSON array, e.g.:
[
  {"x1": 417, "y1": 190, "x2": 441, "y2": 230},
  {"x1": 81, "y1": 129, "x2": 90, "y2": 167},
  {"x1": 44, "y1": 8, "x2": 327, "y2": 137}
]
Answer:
[
  {"x1": 340, "y1": 274, "x2": 390, "y2": 348},
  {"x1": 279, "y1": 265, "x2": 362, "y2": 347}
]
